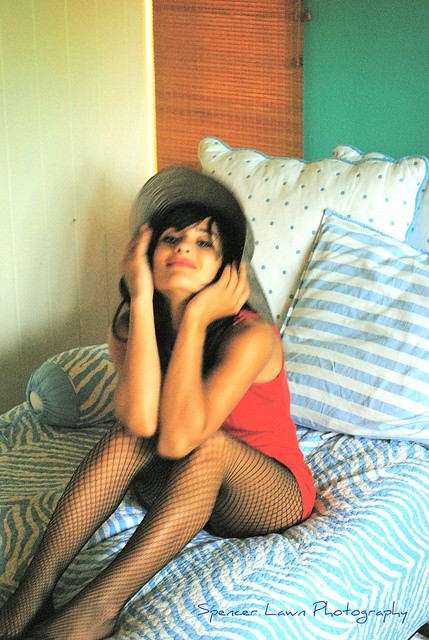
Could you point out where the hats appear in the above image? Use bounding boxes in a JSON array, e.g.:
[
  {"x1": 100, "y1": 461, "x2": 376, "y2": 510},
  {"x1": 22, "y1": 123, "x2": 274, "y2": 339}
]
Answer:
[{"x1": 128, "y1": 162, "x2": 274, "y2": 320}]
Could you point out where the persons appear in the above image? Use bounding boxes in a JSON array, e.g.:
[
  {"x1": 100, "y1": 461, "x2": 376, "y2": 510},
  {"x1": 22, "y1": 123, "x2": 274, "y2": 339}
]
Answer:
[{"x1": 0, "y1": 167, "x2": 316, "y2": 633}]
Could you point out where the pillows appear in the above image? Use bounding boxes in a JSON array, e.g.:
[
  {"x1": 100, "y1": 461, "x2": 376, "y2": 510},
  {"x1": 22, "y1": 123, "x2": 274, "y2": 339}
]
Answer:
[
  {"x1": 279, "y1": 209, "x2": 428, "y2": 444},
  {"x1": 0, "y1": 335, "x2": 117, "y2": 444},
  {"x1": 330, "y1": 145, "x2": 428, "y2": 251},
  {"x1": 199, "y1": 137, "x2": 429, "y2": 327}
]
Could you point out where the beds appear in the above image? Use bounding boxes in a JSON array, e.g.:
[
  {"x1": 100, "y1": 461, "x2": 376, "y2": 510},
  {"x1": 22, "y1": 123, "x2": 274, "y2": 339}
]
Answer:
[{"x1": 0, "y1": 400, "x2": 429, "y2": 640}]
